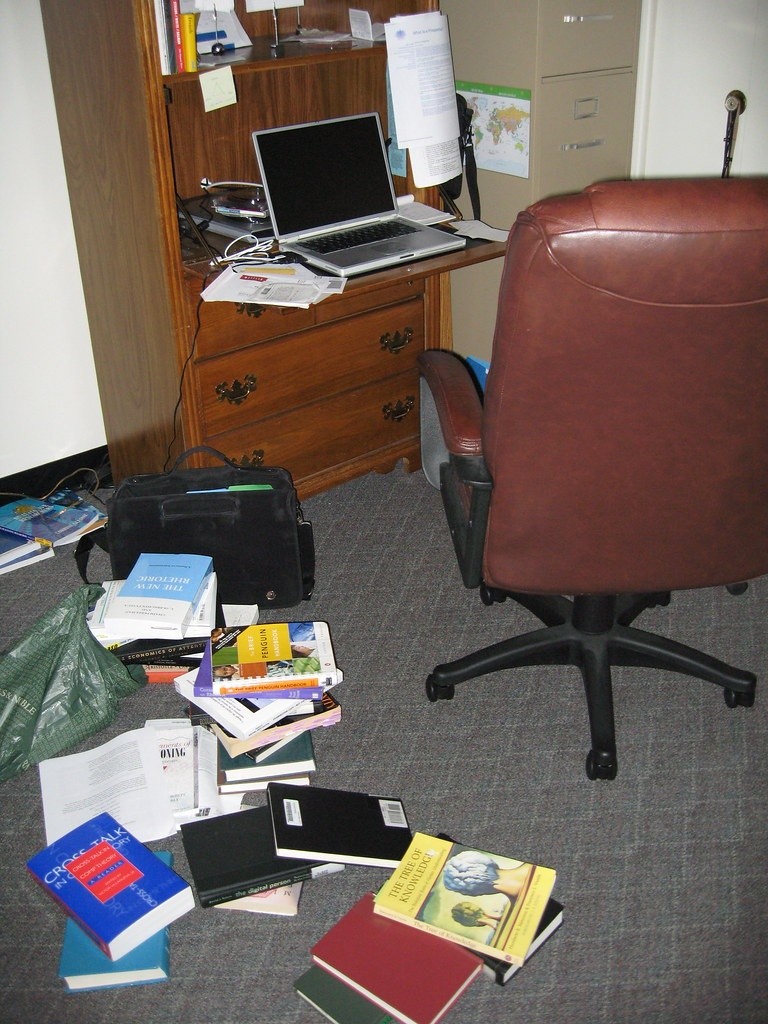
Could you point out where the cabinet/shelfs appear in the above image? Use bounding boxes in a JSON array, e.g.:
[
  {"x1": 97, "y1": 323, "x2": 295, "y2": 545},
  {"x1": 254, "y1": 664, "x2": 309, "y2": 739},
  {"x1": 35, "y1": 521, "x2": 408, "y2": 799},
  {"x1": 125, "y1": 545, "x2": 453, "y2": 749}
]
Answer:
[
  {"x1": 437, "y1": 0, "x2": 640, "y2": 365},
  {"x1": 40, "y1": 0, "x2": 506, "y2": 503}
]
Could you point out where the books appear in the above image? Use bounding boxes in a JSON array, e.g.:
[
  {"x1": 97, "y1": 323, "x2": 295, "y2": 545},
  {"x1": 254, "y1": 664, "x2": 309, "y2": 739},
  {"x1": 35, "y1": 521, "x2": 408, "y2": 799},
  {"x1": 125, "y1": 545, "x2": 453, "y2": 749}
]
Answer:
[
  {"x1": 0, "y1": 490, "x2": 564, "y2": 1024},
  {"x1": 154, "y1": 0, "x2": 198, "y2": 76}
]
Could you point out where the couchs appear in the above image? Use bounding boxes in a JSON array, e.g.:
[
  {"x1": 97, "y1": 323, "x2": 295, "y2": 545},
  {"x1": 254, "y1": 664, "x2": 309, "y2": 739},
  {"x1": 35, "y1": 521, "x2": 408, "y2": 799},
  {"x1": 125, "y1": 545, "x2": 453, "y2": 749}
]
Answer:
[{"x1": 414, "y1": 174, "x2": 768, "y2": 780}]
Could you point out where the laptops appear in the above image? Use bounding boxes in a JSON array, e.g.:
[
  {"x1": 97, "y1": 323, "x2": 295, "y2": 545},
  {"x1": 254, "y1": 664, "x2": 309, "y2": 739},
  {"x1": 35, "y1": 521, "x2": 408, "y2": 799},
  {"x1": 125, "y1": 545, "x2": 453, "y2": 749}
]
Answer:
[{"x1": 251, "y1": 111, "x2": 466, "y2": 279}]
